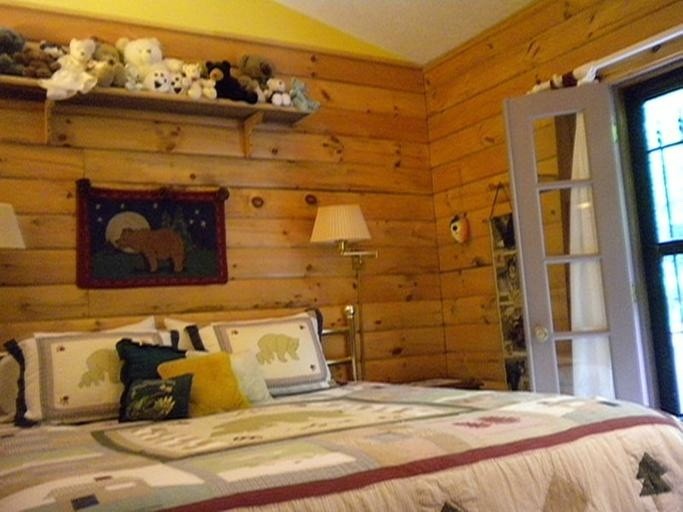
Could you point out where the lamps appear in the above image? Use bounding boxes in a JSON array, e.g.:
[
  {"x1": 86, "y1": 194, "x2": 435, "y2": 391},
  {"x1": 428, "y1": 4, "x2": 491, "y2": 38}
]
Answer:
[
  {"x1": 309, "y1": 203, "x2": 379, "y2": 382},
  {"x1": 0, "y1": 203, "x2": 27, "y2": 251}
]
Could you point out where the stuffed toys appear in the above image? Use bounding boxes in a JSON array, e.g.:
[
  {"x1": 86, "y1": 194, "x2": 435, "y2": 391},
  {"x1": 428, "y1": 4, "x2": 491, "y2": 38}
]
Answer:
[
  {"x1": 17, "y1": 41, "x2": 62, "y2": 79},
  {"x1": 118, "y1": 37, "x2": 186, "y2": 95},
  {"x1": 181, "y1": 63, "x2": 218, "y2": 99},
  {"x1": 47, "y1": 38, "x2": 96, "y2": 101},
  {"x1": 265, "y1": 80, "x2": 290, "y2": 104},
  {"x1": 234, "y1": 52, "x2": 279, "y2": 101},
  {"x1": 0, "y1": 26, "x2": 27, "y2": 78},
  {"x1": 204, "y1": 60, "x2": 259, "y2": 105},
  {"x1": 165, "y1": 54, "x2": 186, "y2": 82},
  {"x1": 94, "y1": 37, "x2": 129, "y2": 88},
  {"x1": 290, "y1": 75, "x2": 321, "y2": 111}
]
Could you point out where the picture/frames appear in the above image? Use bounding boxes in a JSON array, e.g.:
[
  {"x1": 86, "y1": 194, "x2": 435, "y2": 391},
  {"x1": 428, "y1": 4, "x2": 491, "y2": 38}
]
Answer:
[{"x1": 73, "y1": 179, "x2": 229, "y2": 290}]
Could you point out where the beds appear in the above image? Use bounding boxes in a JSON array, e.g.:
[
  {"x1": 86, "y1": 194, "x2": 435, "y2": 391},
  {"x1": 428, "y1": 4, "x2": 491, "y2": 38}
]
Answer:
[{"x1": 0, "y1": 305, "x2": 683, "y2": 512}]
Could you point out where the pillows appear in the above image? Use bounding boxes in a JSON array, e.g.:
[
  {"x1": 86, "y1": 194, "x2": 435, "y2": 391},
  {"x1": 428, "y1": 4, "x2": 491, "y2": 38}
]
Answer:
[
  {"x1": 3, "y1": 314, "x2": 161, "y2": 423},
  {"x1": 190, "y1": 350, "x2": 274, "y2": 420},
  {"x1": 117, "y1": 336, "x2": 191, "y2": 420},
  {"x1": 200, "y1": 310, "x2": 337, "y2": 402},
  {"x1": 34, "y1": 325, "x2": 167, "y2": 428}
]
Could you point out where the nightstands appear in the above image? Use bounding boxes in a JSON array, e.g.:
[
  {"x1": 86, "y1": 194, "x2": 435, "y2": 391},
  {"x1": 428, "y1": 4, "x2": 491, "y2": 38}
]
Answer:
[{"x1": 404, "y1": 377, "x2": 486, "y2": 392}]
